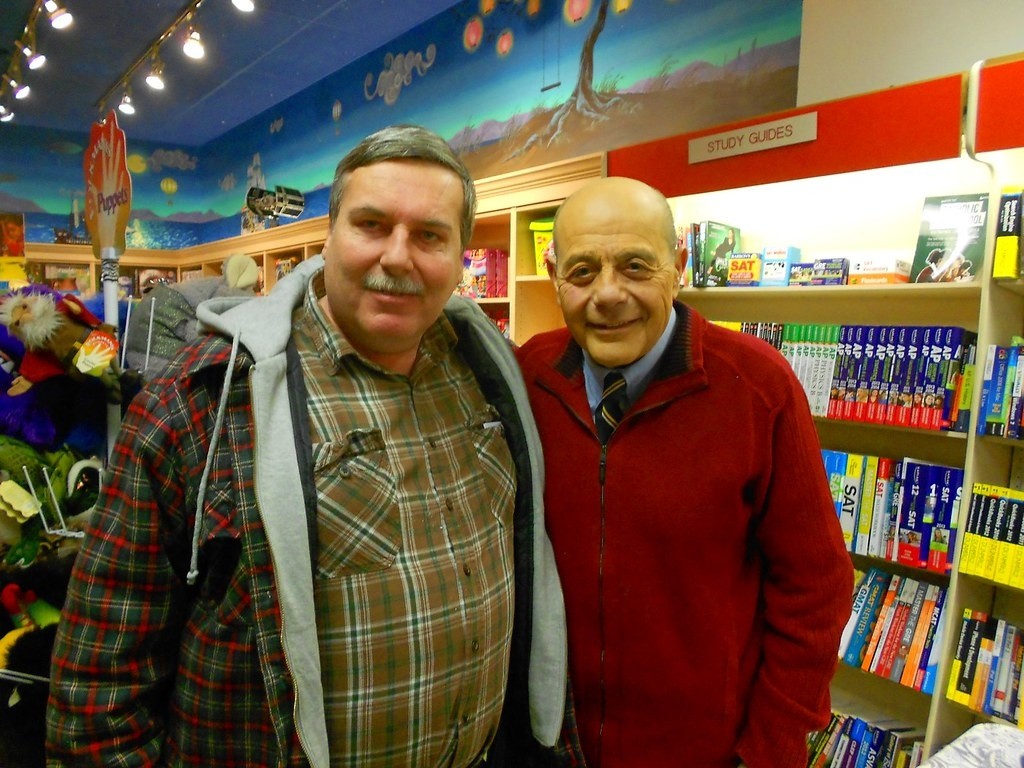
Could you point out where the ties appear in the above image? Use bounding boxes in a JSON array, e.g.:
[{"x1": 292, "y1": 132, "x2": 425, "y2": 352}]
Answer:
[{"x1": 596, "y1": 371, "x2": 627, "y2": 443}]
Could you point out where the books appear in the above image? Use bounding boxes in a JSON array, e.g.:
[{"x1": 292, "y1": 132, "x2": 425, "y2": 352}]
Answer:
[
  {"x1": 908, "y1": 184, "x2": 1024, "y2": 284},
  {"x1": 709, "y1": 320, "x2": 976, "y2": 431},
  {"x1": 451, "y1": 247, "x2": 507, "y2": 298},
  {"x1": 675, "y1": 220, "x2": 742, "y2": 287},
  {"x1": 945, "y1": 607, "x2": 1024, "y2": 731},
  {"x1": 958, "y1": 483, "x2": 1024, "y2": 590},
  {"x1": 975, "y1": 336, "x2": 1024, "y2": 440},
  {"x1": 820, "y1": 448, "x2": 964, "y2": 695},
  {"x1": 806, "y1": 687, "x2": 924, "y2": 768}
]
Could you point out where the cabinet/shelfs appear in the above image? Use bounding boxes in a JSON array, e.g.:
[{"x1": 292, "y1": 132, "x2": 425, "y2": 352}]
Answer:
[
  {"x1": 600, "y1": 51, "x2": 1024, "y2": 768},
  {"x1": 177, "y1": 150, "x2": 605, "y2": 346},
  {"x1": 24, "y1": 242, "x2": 178, "y2": 302}
]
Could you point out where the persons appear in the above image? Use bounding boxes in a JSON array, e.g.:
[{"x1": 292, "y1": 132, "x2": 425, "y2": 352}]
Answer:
[
  {"x1": 830, "y1": 388, "x2": 838, "y2": 400},
  {"x1": 900, "y1": 392, "x2": 911, "y2": 407},
  {"x1": 706, "y1": 228, "x2": 736, "y2": 275},
  {"x1": 856, "y1": 388, "x2": 868, "y2": 403},
  {"x1": 878, "y1": 390, "x2": 887, "y2": 404},
  {"x1": 923, "y1": 394, "x2": 934, "y2": 408},
  {"x1": 47, "y1": 124, "x2": 566, "y2": 768},
  {"x1": 934, "y1": 396, "x2": 943, "y2": 409},
  {"x1": 528, "y1": 176, "x2": 854, "y2": 768},
  {"x1": 869, "y1": 389, "x2": 878, "y2": 403},
  {"x1": 888, "y1": 391, "x2": 898, "y2": 405},
  {"x1": 913, "y1": 392, "x2": 922, "y2": 408},
  {"x1": 845, "y1": 389, "x2": 854, "y2": 401},
  {"x1": 837, "y1": 388, "x2": 845, "y2": 400}
]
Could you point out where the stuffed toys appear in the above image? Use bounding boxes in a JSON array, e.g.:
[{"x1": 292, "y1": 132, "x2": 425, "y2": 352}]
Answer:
[{"x1": 0, "y1": 283, "x2": 127, "y2": 768}]
